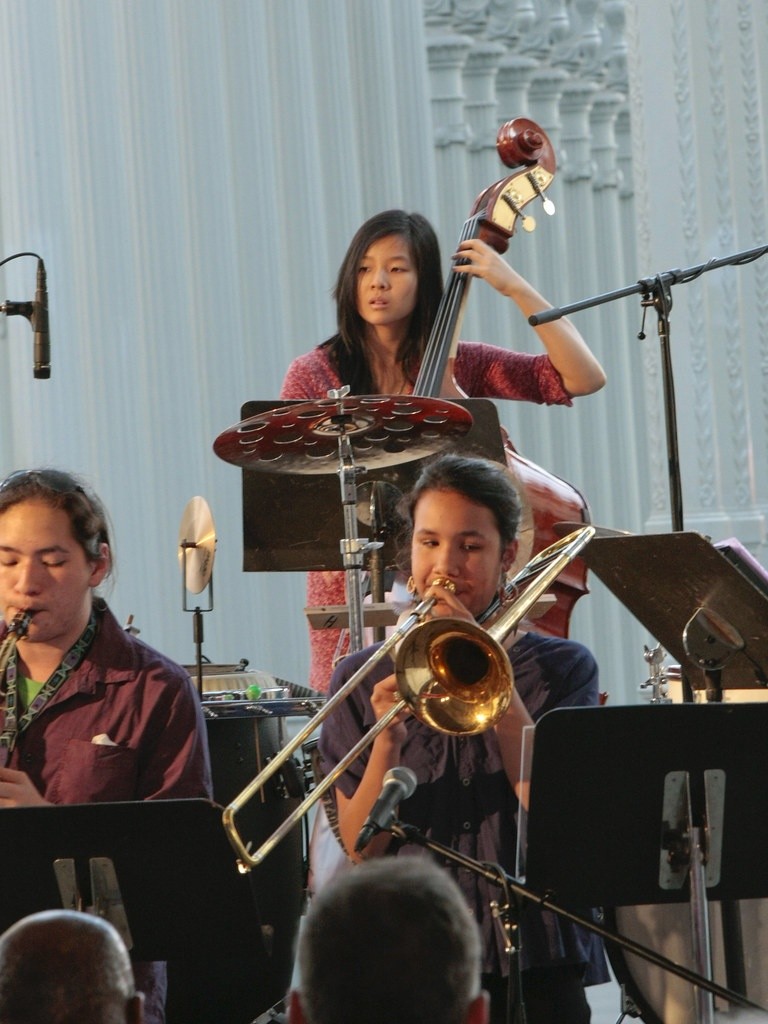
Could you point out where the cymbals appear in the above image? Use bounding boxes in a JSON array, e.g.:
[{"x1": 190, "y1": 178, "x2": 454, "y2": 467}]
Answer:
[{"x1": 211, "y1": 393, "x2": 474, "y2": 477}]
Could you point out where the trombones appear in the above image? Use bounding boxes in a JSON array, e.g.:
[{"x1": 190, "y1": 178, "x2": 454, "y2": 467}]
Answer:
[{"x1": 222, "y1": 524, "x2": 602, "y2": 874}]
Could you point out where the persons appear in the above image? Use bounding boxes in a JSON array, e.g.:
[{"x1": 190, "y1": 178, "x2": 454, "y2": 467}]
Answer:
[
  {"x1": 315, "y1": 455, "x2": 612, "y2": 1024},
  {"x1": 0, "y1": 471, "x2": 214, "y2": 811},
  {"x1": 283, "y1": 210, "x2": 609, "y2": 695},
  {"x1": 287, "y1": 857, "x2": 490, "y2": 1024},
  {"x1": 0, "y1": 910, "x2": 143, "y2": 1024}
]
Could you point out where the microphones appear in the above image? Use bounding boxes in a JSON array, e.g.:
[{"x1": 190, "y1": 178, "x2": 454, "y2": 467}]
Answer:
[
  {"x1": 32, "y1": 259, "x2": 52, "y2": 379},
  {"x1": 354, "y1": 767, "x2": 418, "y2": 854}
]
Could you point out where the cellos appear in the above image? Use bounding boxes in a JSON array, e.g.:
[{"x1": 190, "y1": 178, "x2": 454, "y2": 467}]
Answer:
[{"x1": 360, "y1": 116, "x2": 609, "y2": 738}]
